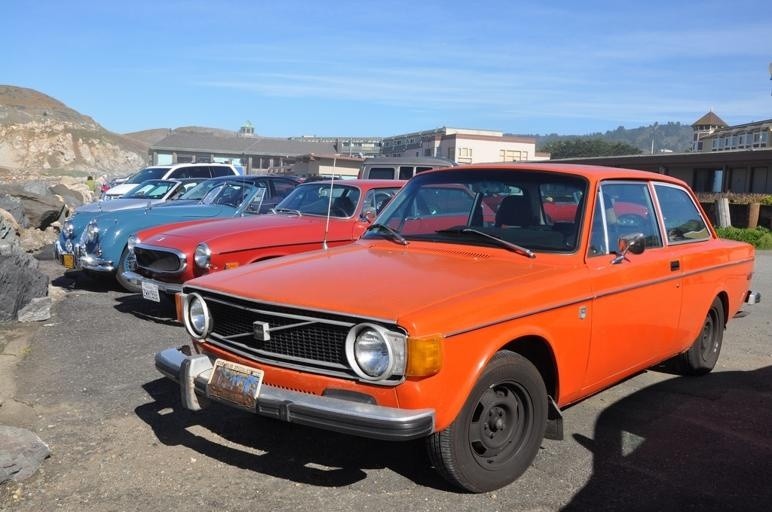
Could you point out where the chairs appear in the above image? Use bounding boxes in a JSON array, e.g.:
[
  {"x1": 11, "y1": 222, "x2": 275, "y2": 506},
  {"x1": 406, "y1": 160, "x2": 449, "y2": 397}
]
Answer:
[
  {"x1": 332, "y1": 195, "x2": 389, "y2": 219},
  {"x1": 490, "y1": 191, "x2": 616, "y2": 251}
]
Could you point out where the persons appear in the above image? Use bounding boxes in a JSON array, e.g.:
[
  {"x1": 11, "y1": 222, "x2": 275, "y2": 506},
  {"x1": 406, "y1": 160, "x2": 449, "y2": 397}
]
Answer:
[
  {"x1": 84, "y1": 175, "x2": 96, "y2": 194},
  {"x1": 99, "y1": 181, "x2": 111, "y2": 200}
]
